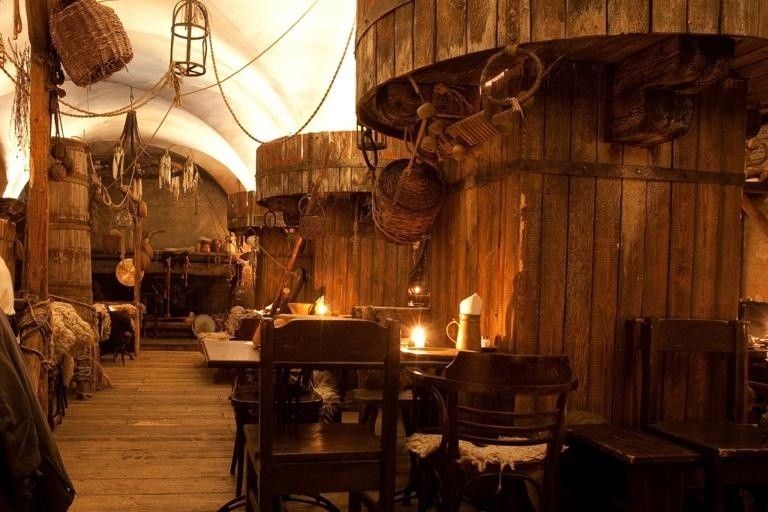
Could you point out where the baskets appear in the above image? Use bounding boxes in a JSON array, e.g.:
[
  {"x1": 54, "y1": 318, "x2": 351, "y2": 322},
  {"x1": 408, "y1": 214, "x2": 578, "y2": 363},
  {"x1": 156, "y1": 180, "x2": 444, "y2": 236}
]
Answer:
[
  {"x1": 371, "y1": 158, "x2": 445, "y2": 242},
  {"x1": 49, "y1": 0, "x2": 133, "y2": 90},
  {"x1": 300, "y1": 215, "x2": 325, "y2": 240}
]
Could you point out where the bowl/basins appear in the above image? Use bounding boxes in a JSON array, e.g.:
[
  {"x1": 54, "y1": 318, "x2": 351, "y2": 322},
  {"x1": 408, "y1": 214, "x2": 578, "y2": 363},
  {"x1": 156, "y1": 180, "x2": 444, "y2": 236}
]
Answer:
[{"x1": 287, "y1": 302, "x2": 314, "y2": 315}]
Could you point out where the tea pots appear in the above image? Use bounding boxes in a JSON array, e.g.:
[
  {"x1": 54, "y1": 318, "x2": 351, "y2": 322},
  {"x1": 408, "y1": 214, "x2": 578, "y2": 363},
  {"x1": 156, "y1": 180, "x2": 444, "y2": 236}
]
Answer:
[{"x1": 445, "y1": 313, "x2": 483, "y2": 352}]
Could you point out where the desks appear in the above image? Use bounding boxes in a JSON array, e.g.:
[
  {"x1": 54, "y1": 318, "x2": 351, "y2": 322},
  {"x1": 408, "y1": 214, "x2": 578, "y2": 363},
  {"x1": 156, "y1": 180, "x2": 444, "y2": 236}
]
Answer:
[{"x1": 198, "y1": 338, "x2": 501, "y2": 498}]
